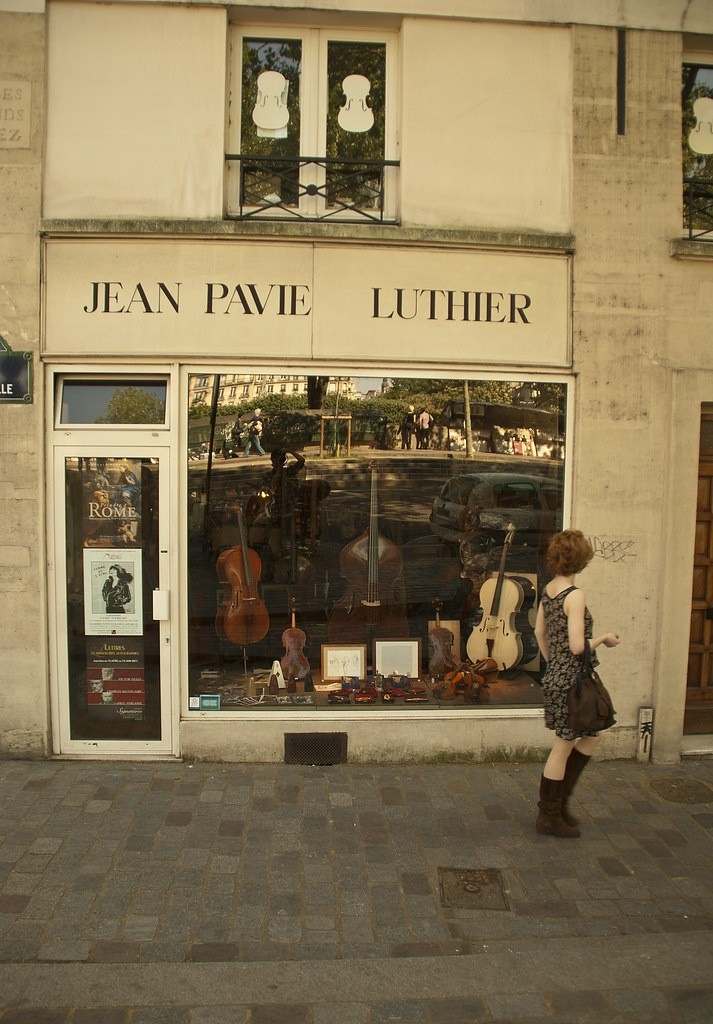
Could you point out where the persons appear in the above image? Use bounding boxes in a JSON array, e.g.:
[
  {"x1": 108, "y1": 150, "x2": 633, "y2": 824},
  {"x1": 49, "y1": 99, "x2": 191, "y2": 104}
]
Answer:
[
  {"x1": 253, "y1": 446, "x2": 306, "y2": 579},
  {"x1": 504, "y1": 427, "x2": 537, "y2": 457},
  {"x1": 404, "y1": 405, "x2": 433, "y2": 449},
  {"x1": 230, "y1": 408, "x2": 268, "y2": 458},
  {"x1": 111, "y1": 462, "x2": 157, "y2": 558},
  {"x1": 534, "y1": 529, "x2": 621, "y2": 838}
]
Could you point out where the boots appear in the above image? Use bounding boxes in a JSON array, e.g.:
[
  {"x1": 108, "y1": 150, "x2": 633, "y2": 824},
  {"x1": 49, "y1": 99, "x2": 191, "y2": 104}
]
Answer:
[
  {"x1": 536, "y1": 771, "x2": 580, "y2": 839},
  {"x1": 559, "y1": 747, "x2": 592, "y2": 826}
]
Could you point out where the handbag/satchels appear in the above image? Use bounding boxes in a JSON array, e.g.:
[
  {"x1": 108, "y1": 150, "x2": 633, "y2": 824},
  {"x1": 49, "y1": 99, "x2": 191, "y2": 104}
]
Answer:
[
  {"x1": 567, "y1": 639, "x2": 617, "y2": 732},
  {"x1": 428, "y1": 414, "x2": 433, "y2": 427},
  {"x1": 231, "y1": 419, "x2": 243, "y2": 433},
  {"x1": 251, "y1": 426, "x2": 259, "y2": 435}
]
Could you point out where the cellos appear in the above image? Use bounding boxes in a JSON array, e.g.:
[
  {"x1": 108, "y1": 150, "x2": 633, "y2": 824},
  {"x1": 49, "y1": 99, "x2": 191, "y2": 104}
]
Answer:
[
  {"x1": 216, "y1": 498, "x2": 270, "y2": 673},
  {"x1": 464, "y1": 523, "x2": 525, "y2": 672},
  {"x1": 273, "y1": 514, "x2": 314, "y2": 587},
  {"x1": 325, "y1": 459, "x2": 410, "y2": 668}
]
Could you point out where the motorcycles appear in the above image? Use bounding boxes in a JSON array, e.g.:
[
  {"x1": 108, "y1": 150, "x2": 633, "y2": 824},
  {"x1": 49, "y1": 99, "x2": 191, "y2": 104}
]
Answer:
[{"x1": 245, "y1": 476, "x2": 332, "y2": 540}]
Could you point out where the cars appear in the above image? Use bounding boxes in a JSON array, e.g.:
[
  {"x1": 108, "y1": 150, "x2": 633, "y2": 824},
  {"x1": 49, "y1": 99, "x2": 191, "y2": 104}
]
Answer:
[{"x1": 428, "y1": 472, "x2": 564, "y2": 554}]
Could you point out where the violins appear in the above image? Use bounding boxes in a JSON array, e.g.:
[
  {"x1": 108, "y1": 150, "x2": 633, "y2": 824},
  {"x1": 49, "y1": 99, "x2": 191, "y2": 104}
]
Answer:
[
  {"x1": 433, "y1": 661, "x2": 467, "y2": 700},
  {"x1": 426, "y1": 599, "x2": 458, "y2": 674},
  {"x1": 461, "y1": 658, "x2": 499, "y2": 704},
  {"x1": 280, "y1": 597, "x2": 311, "y2": 680}
]
